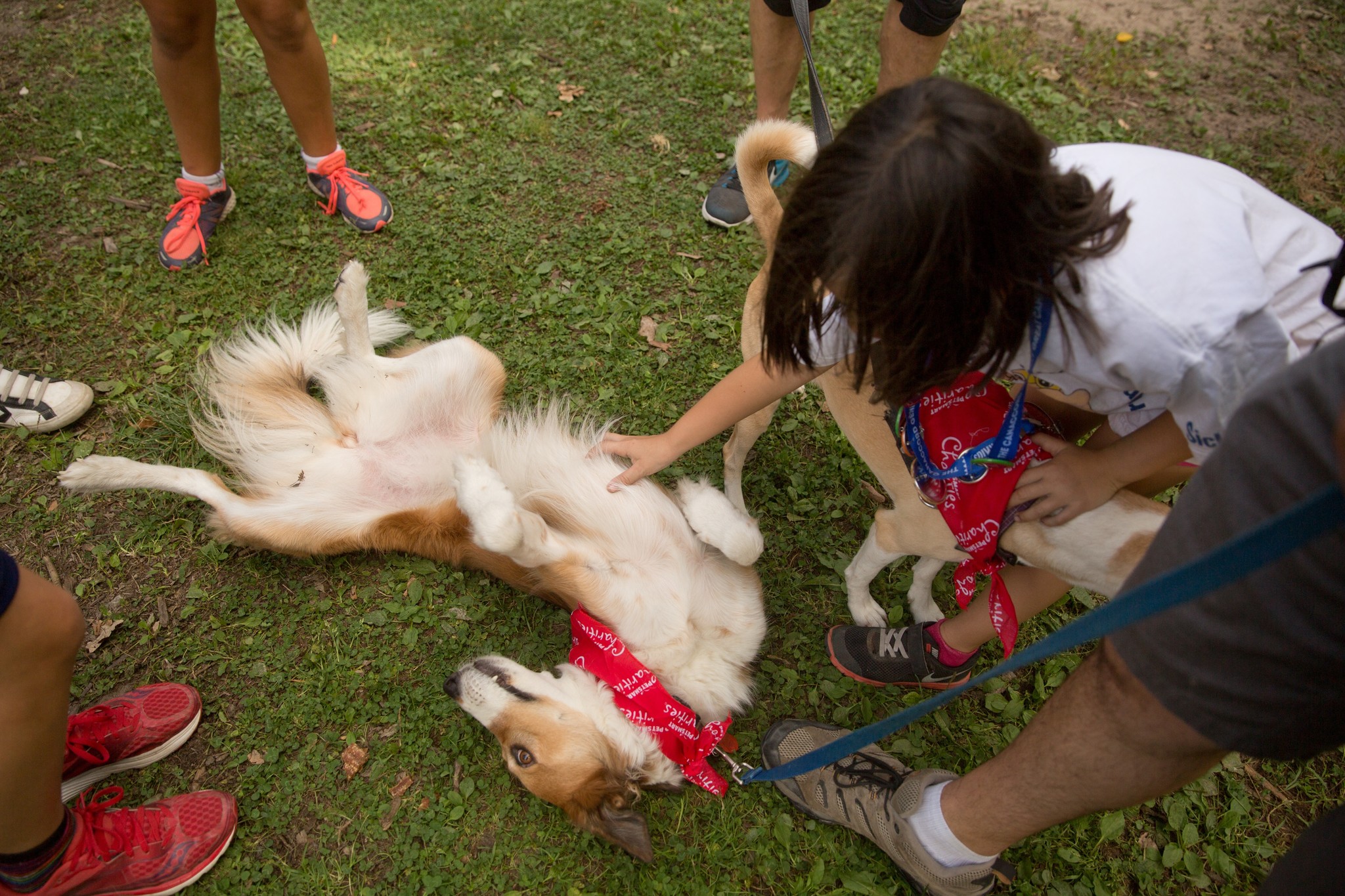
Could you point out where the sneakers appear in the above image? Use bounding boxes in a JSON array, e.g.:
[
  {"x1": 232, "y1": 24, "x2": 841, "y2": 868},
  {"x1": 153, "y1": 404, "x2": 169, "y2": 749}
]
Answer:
[
  {"x1": 824, "y1": 621, "x2": 981, "y2": 691},
  {"x1": 158, "y1": 177, "x2": 237, "y2": 272},
  {"x1": 305, "y1": 148, "x2": 394, "y2": 234},
  {"x1": 61, "y1": 683, "x2": 203, "y2": 804},
  {"x1": 759, "y1": 717, "x2": 1018, "y2": 896},
  {"x1": 0, "y1": 785, "x2": 240, "y2": 896},
  {"x1": 702, "y1": 159, "x2": 790, "y2": 229},
  {"x1": 0, "y1": 360, "x2": 94, "y2": 434}
]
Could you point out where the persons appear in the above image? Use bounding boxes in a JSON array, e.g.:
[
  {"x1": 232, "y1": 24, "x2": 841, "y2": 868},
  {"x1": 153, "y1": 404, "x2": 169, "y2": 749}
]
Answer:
[
  {"x1": 702, "y1": 1, "x2": 973, "y2": 232},
  {"x1": 140, "y1": 0, "x2": 395, "y2": 274},
  {"x1": 0, "y1": 365, "x2": 238, "y2": 896},
  {"x1": 586, "y1": 76, "x2": 1345, "y2": 896}
]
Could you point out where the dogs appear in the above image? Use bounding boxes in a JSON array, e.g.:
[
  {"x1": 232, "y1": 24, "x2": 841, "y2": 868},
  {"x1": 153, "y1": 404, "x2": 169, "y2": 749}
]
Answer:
[
  {"x1": 723, "y1": 116, "x2": 1172, "y2": 628},
  {"x1": 58, "y1": 256, "x2": 766, "y2": 865}
]
